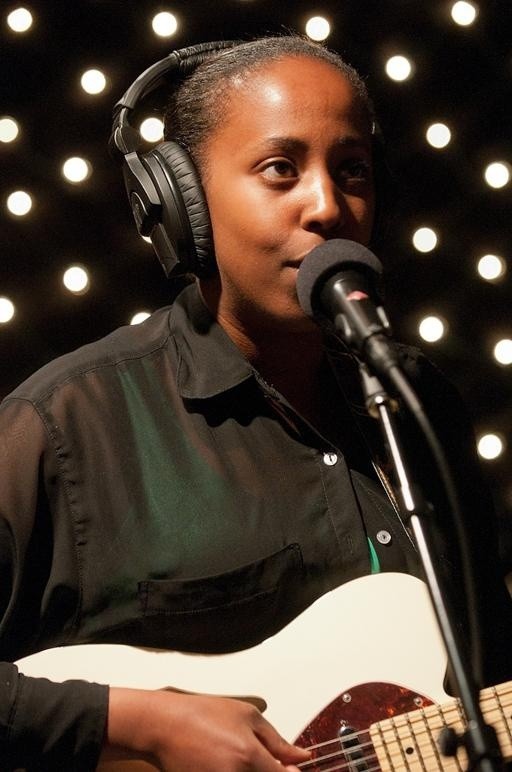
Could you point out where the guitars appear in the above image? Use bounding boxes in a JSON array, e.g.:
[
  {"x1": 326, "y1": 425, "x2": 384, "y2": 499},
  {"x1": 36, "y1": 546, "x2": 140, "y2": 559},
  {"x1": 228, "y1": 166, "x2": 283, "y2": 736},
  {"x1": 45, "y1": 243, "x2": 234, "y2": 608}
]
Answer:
[{"x1": 14, "y1": 571, "x2": 512, "y2": 772}]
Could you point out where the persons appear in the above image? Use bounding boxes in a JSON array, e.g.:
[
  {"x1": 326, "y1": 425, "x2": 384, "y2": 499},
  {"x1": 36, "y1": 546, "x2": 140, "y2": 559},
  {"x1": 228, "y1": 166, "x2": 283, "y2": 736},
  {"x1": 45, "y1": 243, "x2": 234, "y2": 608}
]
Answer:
[{"x1": 0, "y1": 36, "x2": 512, "y2": 772}]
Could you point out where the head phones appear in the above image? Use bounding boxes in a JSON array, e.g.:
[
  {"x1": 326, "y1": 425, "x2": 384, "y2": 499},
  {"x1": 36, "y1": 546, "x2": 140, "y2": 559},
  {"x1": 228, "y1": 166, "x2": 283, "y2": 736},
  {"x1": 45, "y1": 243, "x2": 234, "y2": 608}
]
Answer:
[{"x1": 109, "y1": 39, "x2": 252, "y2": 280}]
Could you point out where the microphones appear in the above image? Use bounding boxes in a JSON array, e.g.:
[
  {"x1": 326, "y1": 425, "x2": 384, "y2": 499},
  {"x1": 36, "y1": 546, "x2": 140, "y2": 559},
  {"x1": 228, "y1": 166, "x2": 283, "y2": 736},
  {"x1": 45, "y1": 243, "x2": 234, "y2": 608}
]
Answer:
[{"x1": 296, "y1": 238, "x2": 398, "y2": 374}]
